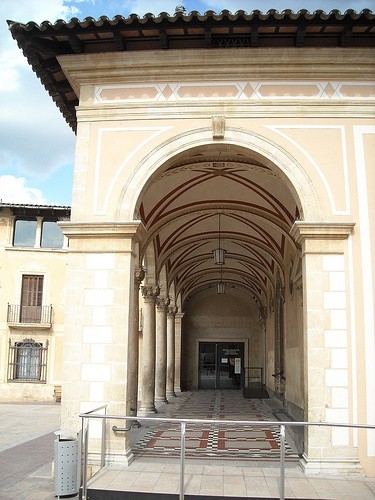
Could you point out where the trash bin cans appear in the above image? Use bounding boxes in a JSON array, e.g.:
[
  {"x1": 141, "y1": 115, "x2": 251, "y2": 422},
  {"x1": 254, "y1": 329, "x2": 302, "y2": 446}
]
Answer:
[{"x1": 52, "y1": 429, "x2": 83, "y2": 500}]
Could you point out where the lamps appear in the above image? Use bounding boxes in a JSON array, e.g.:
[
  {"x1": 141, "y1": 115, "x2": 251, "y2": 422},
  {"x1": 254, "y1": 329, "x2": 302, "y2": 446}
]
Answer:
[
  {"x1": 217, "y1": 264, "x2": 227, "y2": 295},
  {"x1": 211, "y1": 212, "x2": 227, "y2": 265}
]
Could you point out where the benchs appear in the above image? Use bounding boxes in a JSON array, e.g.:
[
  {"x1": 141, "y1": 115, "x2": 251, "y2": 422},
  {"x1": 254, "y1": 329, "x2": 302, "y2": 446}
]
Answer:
[{"x1": 53, "y1": 385, "x2": 61, "y2": 402}]
